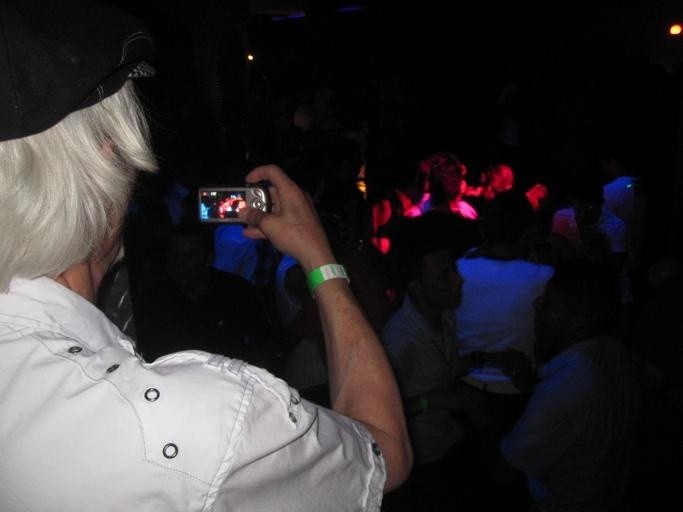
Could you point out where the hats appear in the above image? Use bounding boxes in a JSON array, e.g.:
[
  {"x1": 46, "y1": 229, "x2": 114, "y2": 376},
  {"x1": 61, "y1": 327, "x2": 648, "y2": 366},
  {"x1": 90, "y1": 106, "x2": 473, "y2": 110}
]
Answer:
[{"x1": 1, "y1": 19, "x2": 157, "y2": 140}]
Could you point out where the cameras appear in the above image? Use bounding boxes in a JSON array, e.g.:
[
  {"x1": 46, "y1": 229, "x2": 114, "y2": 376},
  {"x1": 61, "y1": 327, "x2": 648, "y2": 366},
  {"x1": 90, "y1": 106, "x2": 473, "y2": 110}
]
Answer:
[{"x1": 198, "y1": 183, "x2": 272, "y2": 224}]
